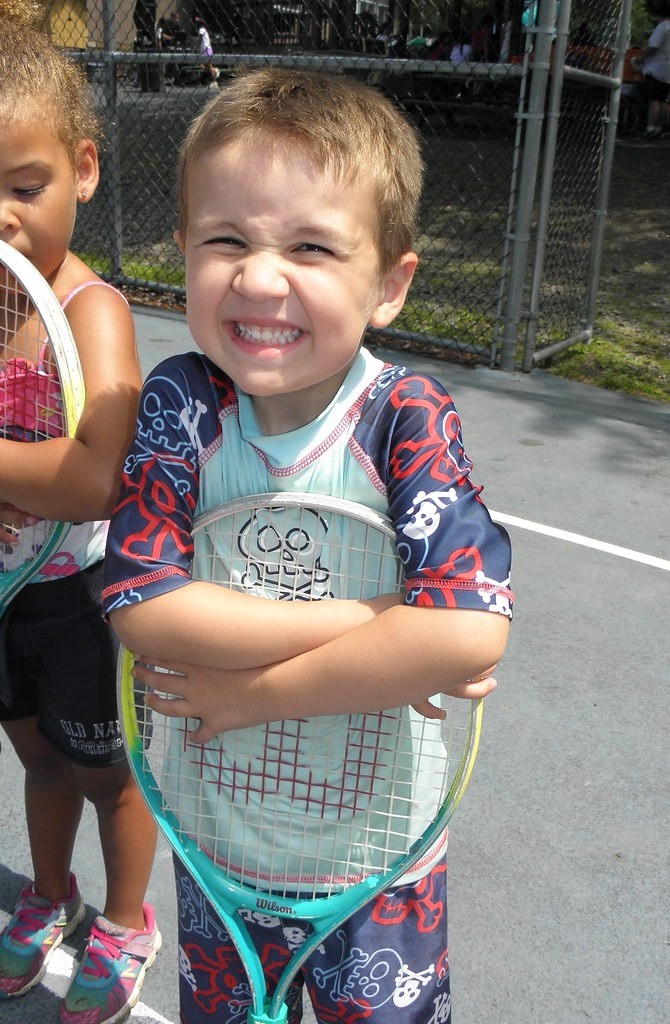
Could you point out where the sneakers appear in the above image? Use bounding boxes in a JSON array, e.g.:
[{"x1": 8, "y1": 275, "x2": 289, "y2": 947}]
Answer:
[
  {"x1": 0, "y1": 871, "x2": 84, "y2": 1000},
  {"x1": 59, "y1": 900, "x2": 165, "y2": 1024}
]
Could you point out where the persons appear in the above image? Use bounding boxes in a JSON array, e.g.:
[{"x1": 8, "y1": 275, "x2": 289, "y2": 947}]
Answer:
[
  {"x1": 155, "y1": 13, "x2": 172, "y2": 53},
  {"x1": 628, "y1": 1, "x2": 670, "y2": 140},
  {"x1": 372, "y1": 24, "x2": 533, "y2": 64},
  {"x1": 163, "y1": 6, "x2": 189, "y2": 52},
  {"x1": 1, "y1": 1, "x2": 169, "y2": 1023},
  {"x1": 89, "y1": 66, "x2": 519, "y2": 1023},
  {"x1": 192, "y1": 14, "x2": 223, "y2": 88}
]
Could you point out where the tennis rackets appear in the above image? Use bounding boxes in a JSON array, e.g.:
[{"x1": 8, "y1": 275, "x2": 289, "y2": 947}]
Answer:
[
  {"x1": 115, "y1": 492, "x2": 484, "y2": 1023},
  {"x1": 0, "y1": 239, "x2": 85, "y2": 618}
]
